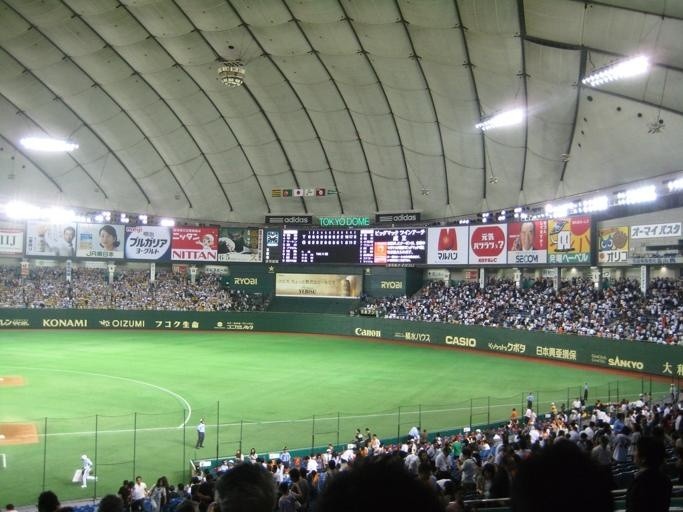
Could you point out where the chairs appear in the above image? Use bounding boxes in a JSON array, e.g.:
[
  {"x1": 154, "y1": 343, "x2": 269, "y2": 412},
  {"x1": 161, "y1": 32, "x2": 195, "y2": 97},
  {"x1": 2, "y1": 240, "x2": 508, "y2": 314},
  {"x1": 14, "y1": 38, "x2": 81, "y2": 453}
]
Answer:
[
  {"x1": 447, "y1": 446, "x2": 683, "y2": 512},
  {"x1": 349, "y1": 278, "x2": 683, "y2": 346}
]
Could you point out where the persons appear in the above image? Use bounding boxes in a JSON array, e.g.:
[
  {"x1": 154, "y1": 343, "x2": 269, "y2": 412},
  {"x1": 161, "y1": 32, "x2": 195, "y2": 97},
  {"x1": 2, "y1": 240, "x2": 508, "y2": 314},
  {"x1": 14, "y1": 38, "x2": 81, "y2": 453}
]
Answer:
[
  {"x1": 97, "y1": 225, "x2": 121, "y2": 251},
  {"x1": 44, "y1": 226, "x2": 75, "y2": 256},
  {"x1": 307, "y1": 381, "x2": 681, "y2": 512},
  {"x1": 195, "y1": 418, "x2": 206, "y2": 450},
  {"x1": 358, "y1": 273, "x2": 682, "y2": 347},
  {"x1": 194, "y1": 234, "x2": 215, "y2": 250},
  {"x1": 510, "y1": 221, "x2": 538, "y2": 250},
  {"x1": 0, "y1": 260, "x2": 262, "y2": 312},
  {"x1": 36, "y1": 446, "x2": 310, "y2": 512}
]
